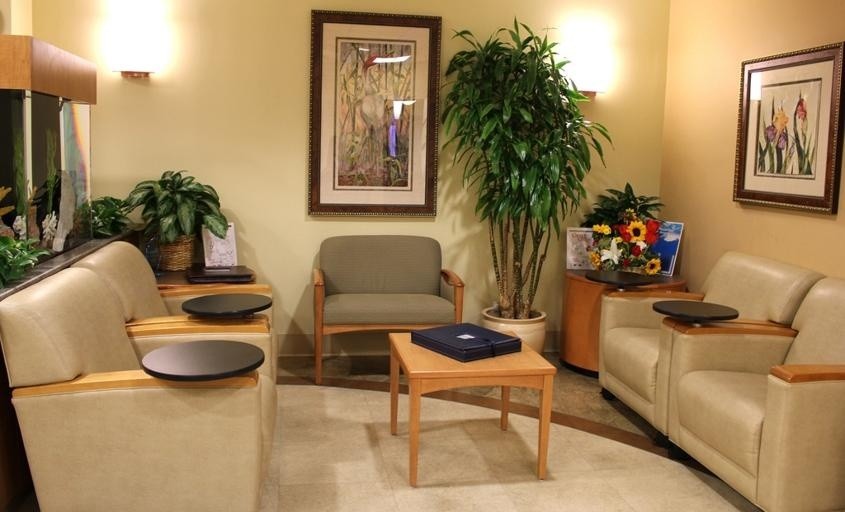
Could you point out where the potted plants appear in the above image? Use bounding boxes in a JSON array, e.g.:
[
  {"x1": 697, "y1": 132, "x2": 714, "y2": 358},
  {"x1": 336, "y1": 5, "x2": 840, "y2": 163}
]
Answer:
[
  {"x1": 442, "y1": 15, "x2": 616, "y2": 356},
  {"x1": 121, "y1": 172, "x2": 227, "y2": 272}
]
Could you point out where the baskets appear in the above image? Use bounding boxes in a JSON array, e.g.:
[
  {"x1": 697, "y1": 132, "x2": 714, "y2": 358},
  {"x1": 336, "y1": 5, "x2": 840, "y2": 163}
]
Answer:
[{"x1": 157, "y1": 236, "x2": 193, "y2": 274}]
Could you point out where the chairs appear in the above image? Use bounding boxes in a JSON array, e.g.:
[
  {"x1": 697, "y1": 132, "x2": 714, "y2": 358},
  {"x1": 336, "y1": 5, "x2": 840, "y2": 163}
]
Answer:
[
  {"x1": 1, "y1": 266, "x2": 277, "y2": 511},
  {"x1": 667, "y1": 279, "x2": 844, "y2": 512},
  {"x1": 597, "y1": 252, "x2": 826, "y2": 447},
  {"x1": 312, "y1": 235, "x2": 463, "y2": 383},
  {"x1": 70, "y1": 242, "x2": 279, "y2": 383}
]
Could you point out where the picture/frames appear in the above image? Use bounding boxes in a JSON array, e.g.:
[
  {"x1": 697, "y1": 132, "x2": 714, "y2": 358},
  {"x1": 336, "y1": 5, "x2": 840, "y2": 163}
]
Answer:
[
  {"x1": 307, "y1": 9, "x2": 440, "y2": 217},
  {"x1": 732, "y1": 31, "x2": 843, "y2": 216}
]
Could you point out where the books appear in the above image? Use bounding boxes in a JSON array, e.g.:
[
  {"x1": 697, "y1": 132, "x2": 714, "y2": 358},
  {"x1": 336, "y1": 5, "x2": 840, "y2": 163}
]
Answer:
[
  {"x1": 563, "y1": 226, "x2": 597, "y2": 270},
  {"x1": 647, "y1": 219, "x2": 684, "y2": 277}
]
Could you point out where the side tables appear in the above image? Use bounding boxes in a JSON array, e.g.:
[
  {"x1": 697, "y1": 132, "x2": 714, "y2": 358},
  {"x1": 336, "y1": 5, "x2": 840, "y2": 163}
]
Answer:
[
  {"x1": 154, "y1": 271, "x2": 256, "y2": 289},
  {"x1": 561, "y1": 266, "x2": 686, "y2": 379}
]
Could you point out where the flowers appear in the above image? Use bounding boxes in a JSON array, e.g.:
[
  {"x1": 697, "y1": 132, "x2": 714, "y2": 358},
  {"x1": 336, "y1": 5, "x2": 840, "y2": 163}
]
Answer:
[{"x1": 580, "y1": 180, "x2": 664, "y2": 276}]
